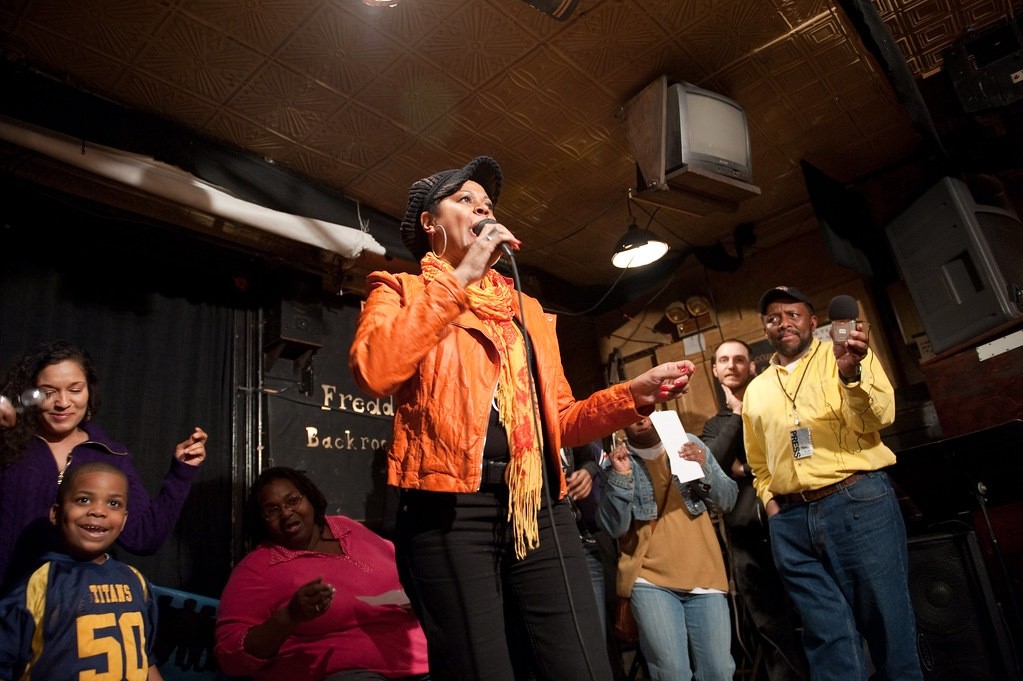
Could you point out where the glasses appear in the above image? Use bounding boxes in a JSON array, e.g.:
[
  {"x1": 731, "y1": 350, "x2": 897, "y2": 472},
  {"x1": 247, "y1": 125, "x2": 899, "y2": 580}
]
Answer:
[{"x1": 262, "y1": 492, "x2": 304, "y2": 521}]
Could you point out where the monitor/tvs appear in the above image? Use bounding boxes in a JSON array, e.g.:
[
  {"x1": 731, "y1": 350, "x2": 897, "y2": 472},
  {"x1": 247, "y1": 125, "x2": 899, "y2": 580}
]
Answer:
[{"x1": 663, "y1": 81, "x2": 753, "y2": 185}]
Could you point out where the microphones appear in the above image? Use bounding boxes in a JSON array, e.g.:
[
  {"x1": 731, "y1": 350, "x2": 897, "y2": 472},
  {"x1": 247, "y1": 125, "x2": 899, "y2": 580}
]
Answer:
[{"x1": 476, "y1": 218, "x2": 514, "y2": 260}]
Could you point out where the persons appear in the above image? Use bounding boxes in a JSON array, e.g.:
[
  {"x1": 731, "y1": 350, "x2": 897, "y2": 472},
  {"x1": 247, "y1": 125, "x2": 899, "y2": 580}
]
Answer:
[
  {"x1": 0, "y1": 461, "x2": 167, "y2": 681},
  {"x1": 213, "y1": 466, "x2": 432, "y2": 681},
  {"x1": 347, "y1": 155, "x2": 695, "y2": 681},
  {"x1": 563, "y1": 337, "x2": 809, "y2": 681},
  {"x1": 743, "y1": 285, "x2": 928, "y2": 681},
  {"x1": 1, "y1": 338, "x2": 208, "y2": 601}
]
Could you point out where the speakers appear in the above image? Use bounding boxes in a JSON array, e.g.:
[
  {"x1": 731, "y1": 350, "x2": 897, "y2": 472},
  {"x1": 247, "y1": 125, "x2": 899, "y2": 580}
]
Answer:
[
  {"x1": 884, "y1": 172, "x2": 1023, "y2": 354},
  {"x1": 261, "y1": 264, "x2": 324, "y2": 359},
  {"x1": 905, "y1": 528, "x2": 1019, "y2": 681}
]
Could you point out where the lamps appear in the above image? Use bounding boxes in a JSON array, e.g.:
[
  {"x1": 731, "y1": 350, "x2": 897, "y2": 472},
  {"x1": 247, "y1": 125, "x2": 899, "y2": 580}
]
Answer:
[
  {"x1": 610, "y1": 198, "x2": 723, "y2": 270},
  {"x1": 665, "y1": 294, "x2": 719, "y2": 340}
]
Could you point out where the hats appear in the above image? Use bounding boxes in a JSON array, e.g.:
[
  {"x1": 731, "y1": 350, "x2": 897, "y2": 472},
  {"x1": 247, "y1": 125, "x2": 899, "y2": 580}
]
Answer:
[
  {"x1": 759, "y1": 286, "x2": 816, "y2": 316},
  {"x1": 401, "y1": 155, "x2": 501, "y2": 262}
]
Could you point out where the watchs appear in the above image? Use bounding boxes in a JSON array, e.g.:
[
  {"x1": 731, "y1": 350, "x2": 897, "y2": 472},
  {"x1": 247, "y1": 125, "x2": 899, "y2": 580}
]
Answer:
[{"x1": 838, "y1": 364, "x2": 863, "y2": 386}]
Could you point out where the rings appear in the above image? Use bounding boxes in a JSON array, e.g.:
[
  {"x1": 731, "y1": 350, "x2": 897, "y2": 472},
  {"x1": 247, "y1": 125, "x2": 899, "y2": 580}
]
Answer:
[
  {"x1": 485, "y1": 233, "x2": 493, "y2": 240},
  {"x1": 697, "y1": 449, "x2": 701, "y2": 455},
  {"x1": 314, "y1": 604, "x2": 321, "y2": 611}
]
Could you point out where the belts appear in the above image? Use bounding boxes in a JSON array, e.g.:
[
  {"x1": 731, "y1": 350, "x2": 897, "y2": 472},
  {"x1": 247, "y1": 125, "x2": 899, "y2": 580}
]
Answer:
[{"x1": 784, "y1": 466, "x2": 872, "y2": 504}]
